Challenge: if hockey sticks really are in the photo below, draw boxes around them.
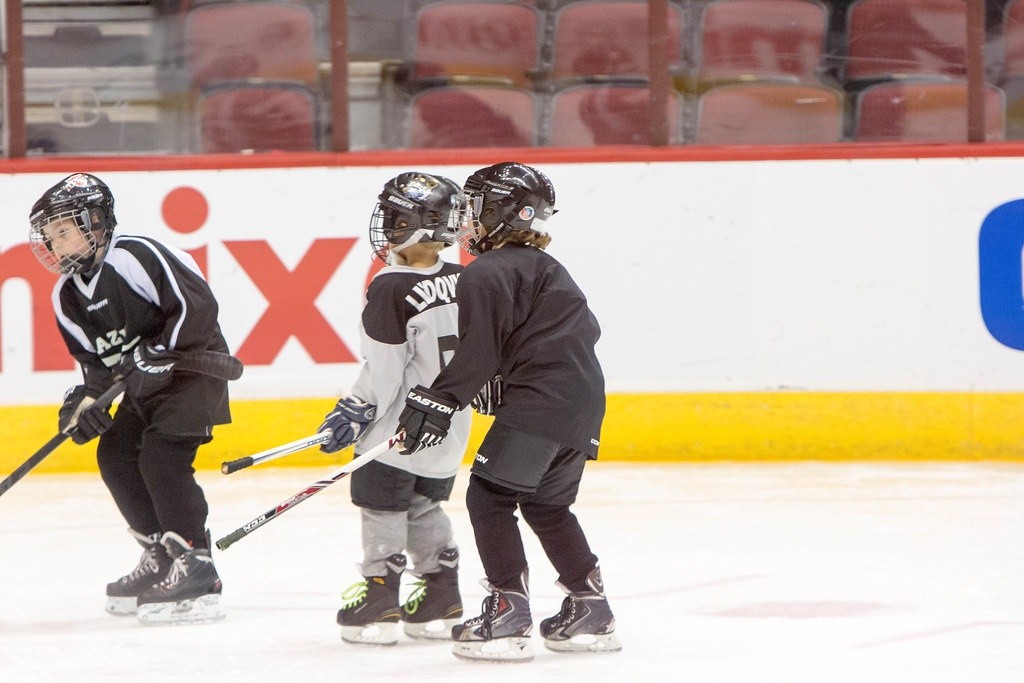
[221,431,331,474]
[214,434,404,548]
[0,349,242,498]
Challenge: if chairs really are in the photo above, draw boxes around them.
[168,0,1024,155]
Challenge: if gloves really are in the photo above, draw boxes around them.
[110,339,176,399]
[318,397,378,454]
[395,383,460,456]
[58,382,114,444]
[470,376,504,416]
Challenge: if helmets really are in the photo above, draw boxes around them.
[368,170,467,266]
[29,173,114,276]
[450,163,556,256]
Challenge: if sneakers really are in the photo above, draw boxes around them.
[450,569,534,661]
[335,553,408,646]
[105,528,176,616]
[398,548,464,640]
[540,564,622,650]
[136,526,226,626]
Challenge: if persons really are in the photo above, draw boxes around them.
[29,174,232,624]
[318,172,465,644]
[396,161,622,663]
